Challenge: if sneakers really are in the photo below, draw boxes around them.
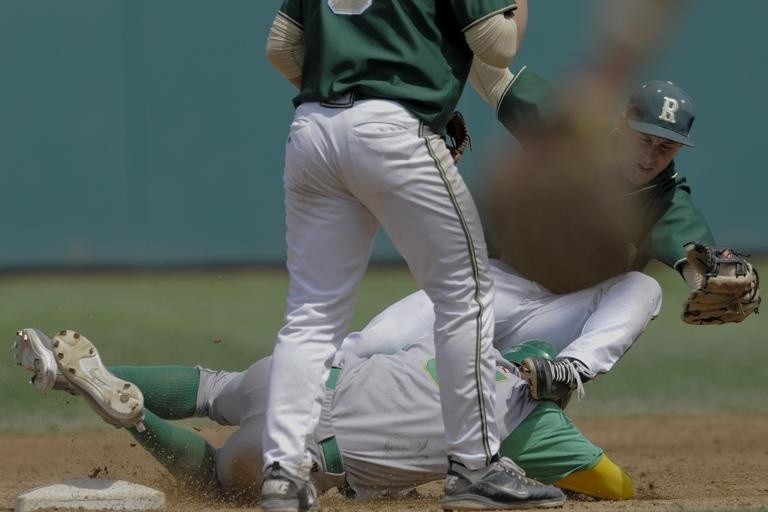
[259,460,317,511]
[522,357,596,401]
[51,329,146,433]
[13,327,70,394]
[441,454,567,512]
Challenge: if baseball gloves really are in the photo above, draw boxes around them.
[673,240,762,326]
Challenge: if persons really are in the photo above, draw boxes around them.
[340,52,759,400]
[258,0,568,512]
[12,327,636,499]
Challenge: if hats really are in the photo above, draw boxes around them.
[626,80,695,148]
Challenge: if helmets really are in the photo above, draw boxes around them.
[502,338,572,409]
